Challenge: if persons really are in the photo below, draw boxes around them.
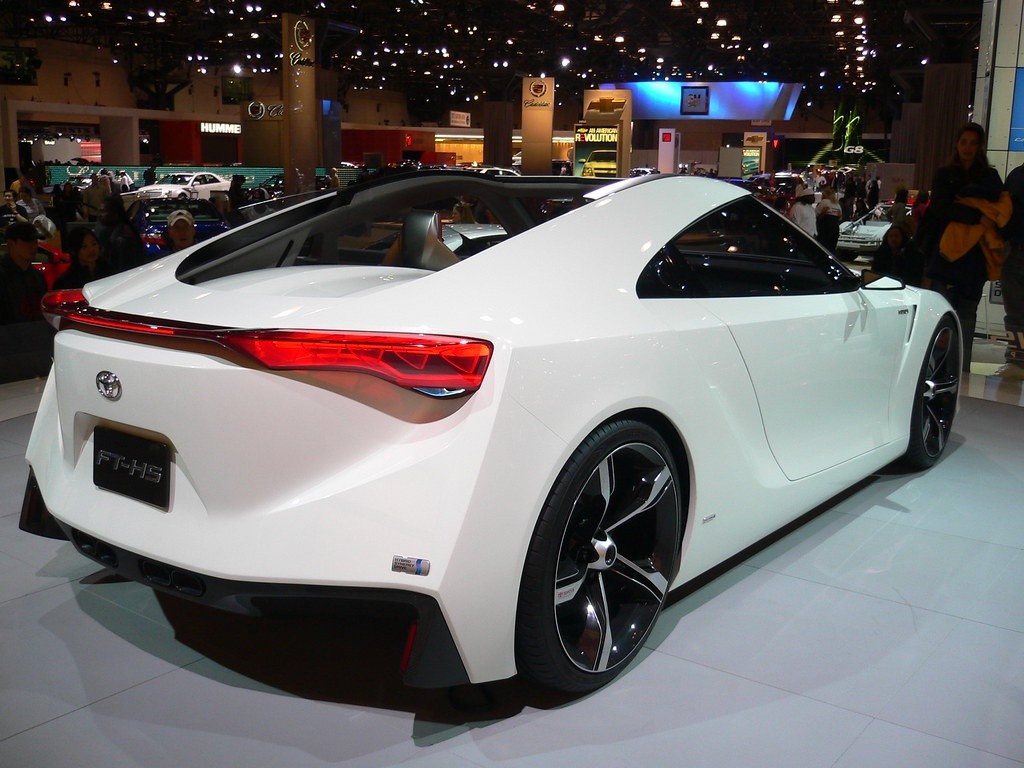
[912,123,1002,373]
[1000,166,1024,364]
[0,165,931,383]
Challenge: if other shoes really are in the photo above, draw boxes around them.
[1000,363,1024,380]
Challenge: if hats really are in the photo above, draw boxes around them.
[5,222,46,241]
[799,189,814,196]
[167,209,193,226]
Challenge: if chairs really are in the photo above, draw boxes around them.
[363,215,483,257]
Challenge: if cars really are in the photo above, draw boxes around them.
[833,201,917,264]
[465,165,522,178]
[631,167,657,177]
[577,149,618,180]
[722,170,808,211]
[134,172,233,201]
[1,170,332,210]
[127,197,231,260]
[28,239,74,289]
[333,204,511,266]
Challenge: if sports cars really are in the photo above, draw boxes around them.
[14,163,968,704]
